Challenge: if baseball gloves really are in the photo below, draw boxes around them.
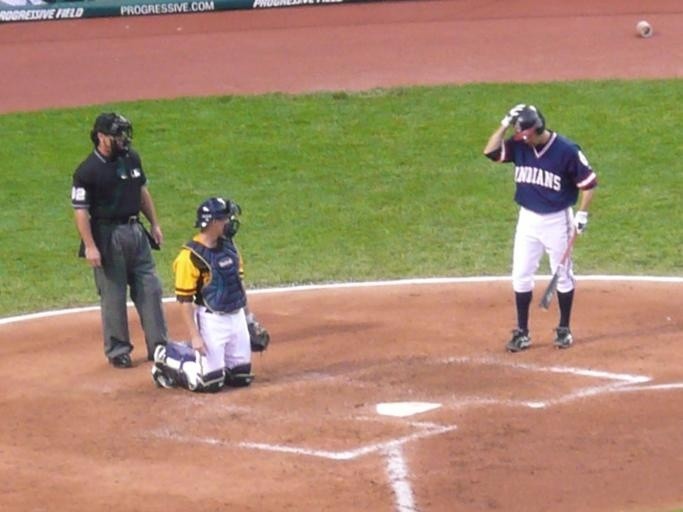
[246,318,268,352]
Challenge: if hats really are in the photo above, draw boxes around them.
[94,112,125,135]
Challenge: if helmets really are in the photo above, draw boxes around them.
[194,198,236,229]
[512,106,546,143]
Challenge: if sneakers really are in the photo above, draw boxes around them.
[508,326,533,351]
[553,326,574,349]
[106,350,133,370]
[152,344,168,388]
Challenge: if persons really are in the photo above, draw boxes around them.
[481,100,598,356]
[148,195,270,396]
[67,109,171,372]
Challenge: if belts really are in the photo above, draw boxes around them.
[92,216,140,224]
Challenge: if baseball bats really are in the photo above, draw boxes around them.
[538,227,579,312]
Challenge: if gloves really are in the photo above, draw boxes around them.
[572,210,588,231]
[500,102,526,127]
